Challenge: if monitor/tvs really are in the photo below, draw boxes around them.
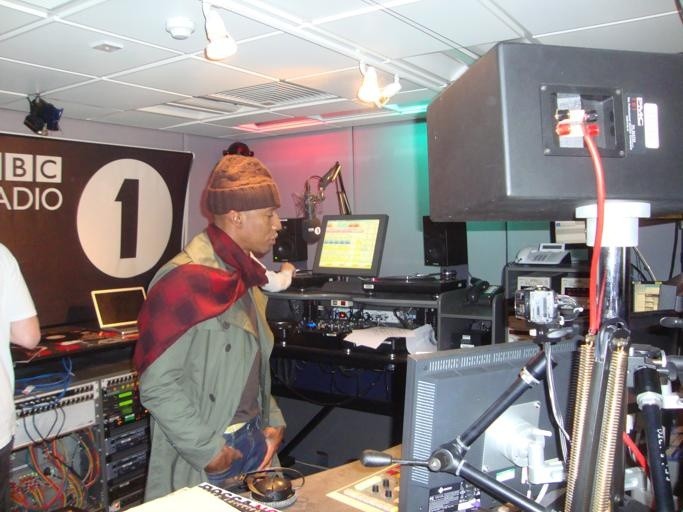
[312,214,388,292]
[398,339,578,512]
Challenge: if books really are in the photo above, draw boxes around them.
[122,480,282,512]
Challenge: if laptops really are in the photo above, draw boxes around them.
[91,287,147,334]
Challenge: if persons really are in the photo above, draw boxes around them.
[135,153,289,503]
[249,251,295,293]
[0,242,41,512]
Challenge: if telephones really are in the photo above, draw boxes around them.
[467,280,503,305]
[514,242,571,267]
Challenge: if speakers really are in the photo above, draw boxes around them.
[423,216,468,266]
[273,218,308,262]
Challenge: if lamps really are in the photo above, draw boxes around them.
[24,95,64,137]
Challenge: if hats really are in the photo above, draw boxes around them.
[206,154,280,215]
